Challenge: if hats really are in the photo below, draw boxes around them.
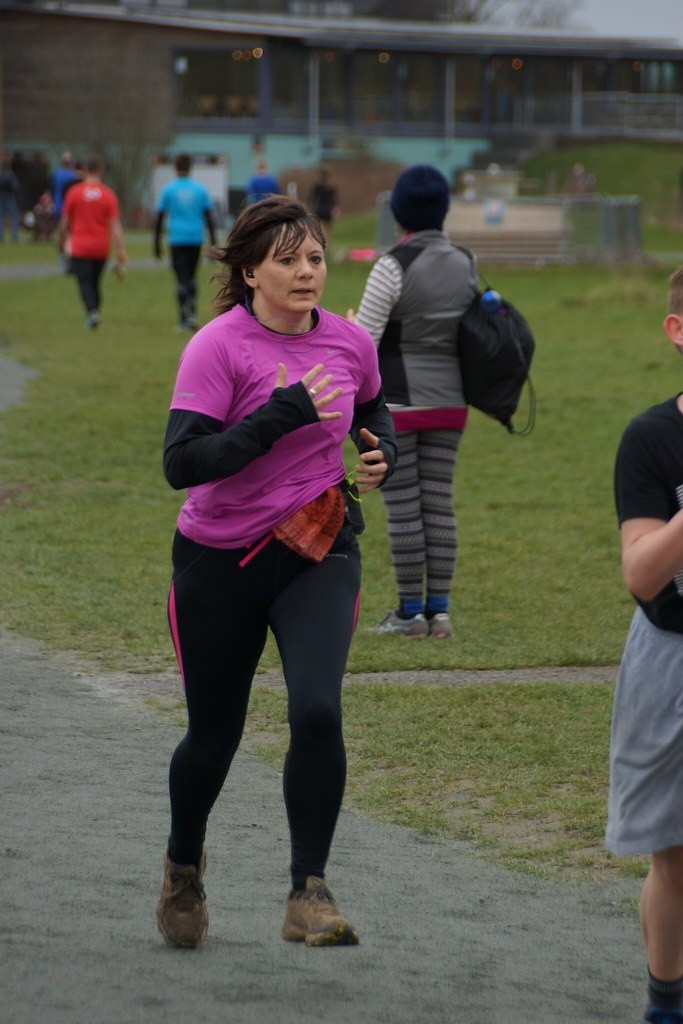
[390,166,449,231]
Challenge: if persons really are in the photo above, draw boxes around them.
[606,264,683,1024]
[347,166,482,638]
[0,151,127,330]
[155,196,400,951]
[246,159,280,206]
[152,149,230,332]
[304,170,341,249]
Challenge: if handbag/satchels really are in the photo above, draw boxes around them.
[455,260,536,437]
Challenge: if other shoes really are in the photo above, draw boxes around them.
[87,314,98,328]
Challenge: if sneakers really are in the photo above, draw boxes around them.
[282,875,359,946]
[367,607,428,639]
[428,613,453,638]
[157,847,209,949]
